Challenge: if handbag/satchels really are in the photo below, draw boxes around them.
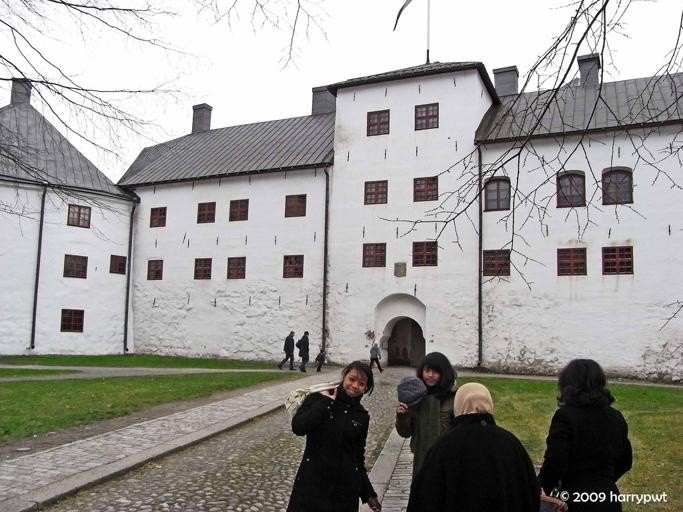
[536,471,570,512]
[284,379,342,418]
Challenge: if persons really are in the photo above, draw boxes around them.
[313,348,325,372]
[394,350,458,483]
[536,358,632,512]
[284,359,382,512]
[276,330,295,370]
[367,342,384,375]
[297,330,309,373]
[405,382,541,511]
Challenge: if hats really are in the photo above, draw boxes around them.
[397,376,429,407]
